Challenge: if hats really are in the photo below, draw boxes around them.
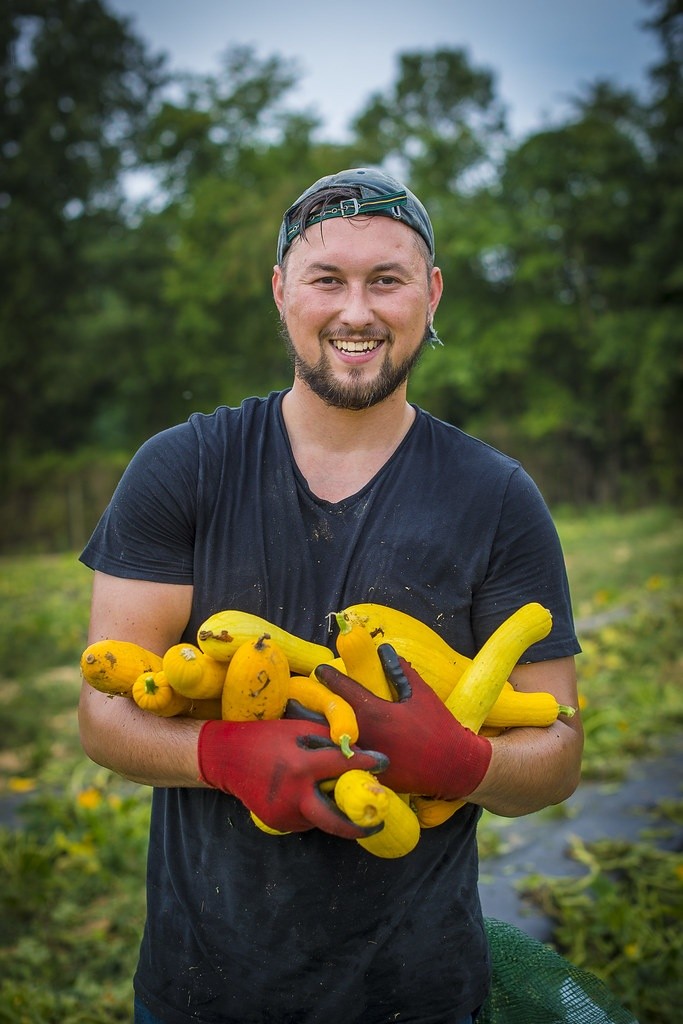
[276,168,435,340]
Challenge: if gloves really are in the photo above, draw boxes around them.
[198,718,387,839]
[282,645,491,801]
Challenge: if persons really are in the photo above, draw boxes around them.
[77,163,585,1022]
